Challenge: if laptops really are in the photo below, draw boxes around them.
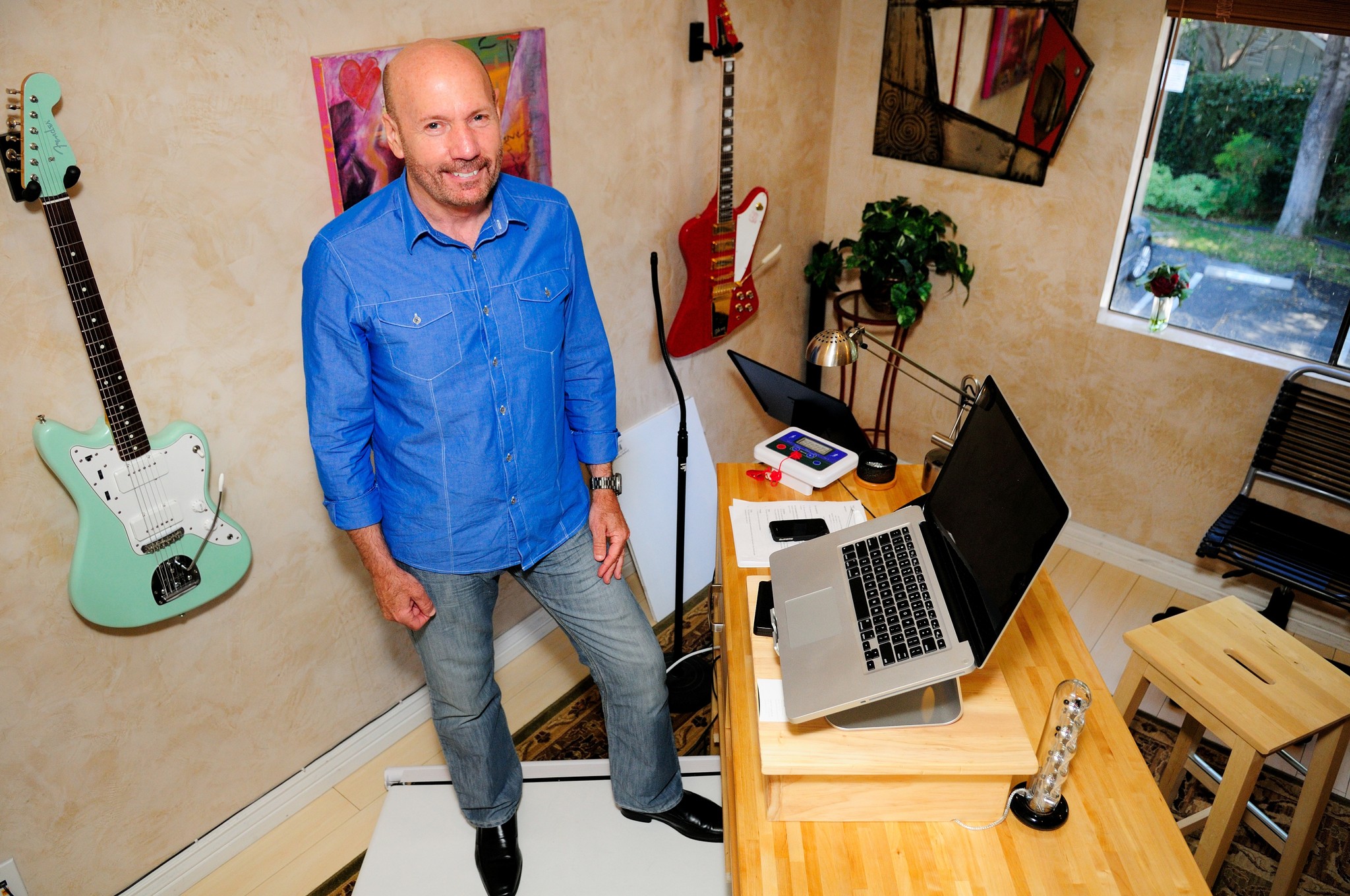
[768,374,1073,729]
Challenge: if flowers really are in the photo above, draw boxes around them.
[1135,261,1190,311]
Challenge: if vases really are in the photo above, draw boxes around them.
[1148,295,1175,332]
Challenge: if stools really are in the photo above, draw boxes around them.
[1111,592,1348,896]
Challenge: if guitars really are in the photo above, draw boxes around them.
[666,0,771,357]
[1,72,253,628]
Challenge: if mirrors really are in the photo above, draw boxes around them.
[870,0,1096,189]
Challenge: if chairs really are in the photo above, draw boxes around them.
[1145,365,1350,676]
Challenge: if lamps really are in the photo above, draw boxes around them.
[805,317,987,496]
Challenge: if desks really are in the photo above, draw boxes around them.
[713,461,1210,896]
[831,293,925,458]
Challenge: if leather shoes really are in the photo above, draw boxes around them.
[620,789,723,844]
[474,813,523,896]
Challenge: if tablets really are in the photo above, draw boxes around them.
[726,350,870,455]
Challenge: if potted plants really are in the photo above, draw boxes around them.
[800,196,976,333]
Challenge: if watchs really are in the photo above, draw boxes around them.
[588,472,623,498]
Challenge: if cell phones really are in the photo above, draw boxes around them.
[769,518,829,542]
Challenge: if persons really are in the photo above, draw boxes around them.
[300,38,723,896]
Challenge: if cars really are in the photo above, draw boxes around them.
[1115,213,1153,287]
